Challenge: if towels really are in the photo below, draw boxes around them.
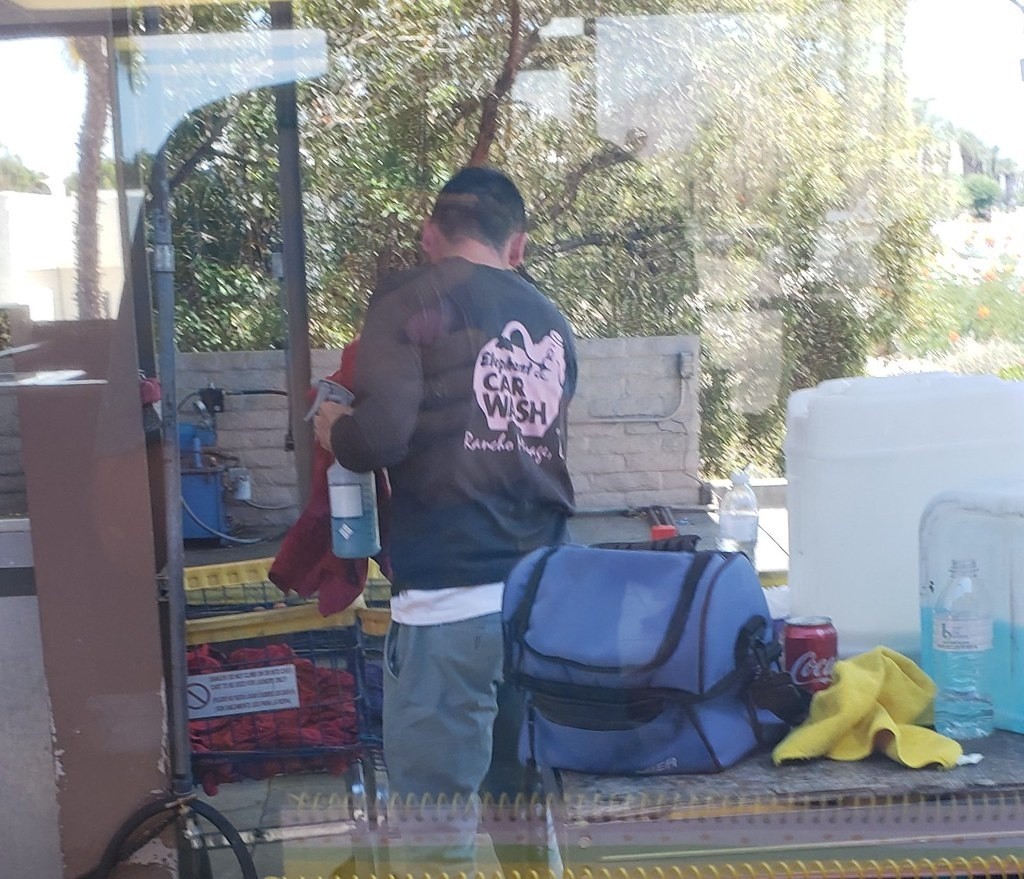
[187,643,358,795]
[268,340,395,614]
[773,644,983,771]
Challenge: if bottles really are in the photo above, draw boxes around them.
[716,472,760,566]
[327,467,380,559]
[933,560,996,740]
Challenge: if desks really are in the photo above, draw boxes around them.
[530,726,1024,879]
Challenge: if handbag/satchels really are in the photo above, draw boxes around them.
[500,545,813,777]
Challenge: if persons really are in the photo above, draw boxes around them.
[313,166,577,879]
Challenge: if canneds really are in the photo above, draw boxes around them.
[783,617,836,696]
